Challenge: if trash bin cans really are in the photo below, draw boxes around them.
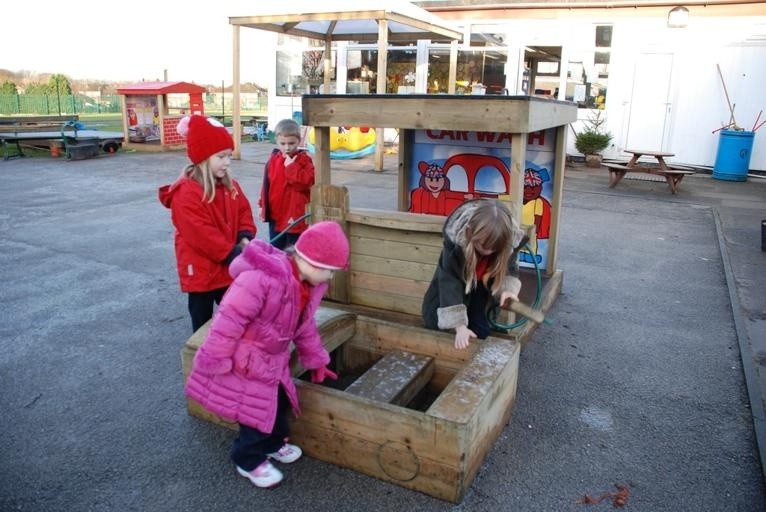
[711,129,755,182]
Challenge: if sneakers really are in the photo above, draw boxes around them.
[265,443,303,464]
[236,460,284,488]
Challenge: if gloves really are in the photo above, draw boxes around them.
[311,367,338,384]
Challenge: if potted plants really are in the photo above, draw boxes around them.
[575,132,613,168]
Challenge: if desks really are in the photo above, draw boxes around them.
[609,149,678,195]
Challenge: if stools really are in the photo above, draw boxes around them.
[600,159,695,195]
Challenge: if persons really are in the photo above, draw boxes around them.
[422,196,531,351]
[254,118,316,247]
[159,113,257,333]
[183,219,349,489]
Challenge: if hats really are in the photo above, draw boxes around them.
[294,221,350,272]
[176,115,234,164]
